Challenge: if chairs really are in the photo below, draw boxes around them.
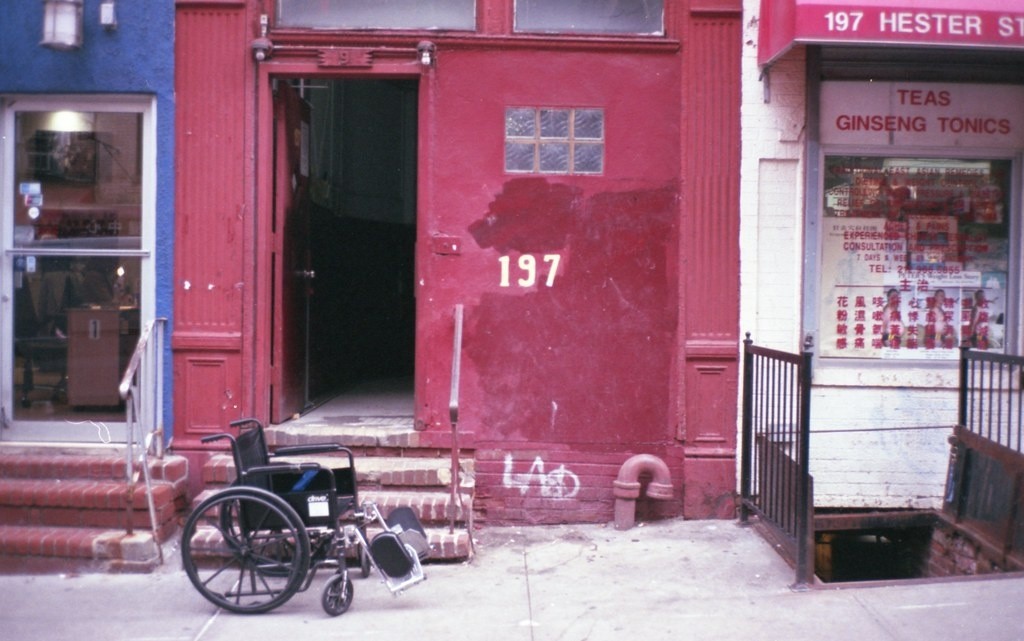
[16,270,107,409]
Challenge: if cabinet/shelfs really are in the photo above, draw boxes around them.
[63,303,139,411]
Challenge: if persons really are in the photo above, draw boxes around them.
[927,290,947,347]
[969,290,990,348]
[884,289,903,347]
[49,244,132,346]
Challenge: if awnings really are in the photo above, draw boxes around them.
[757,0,1024,102]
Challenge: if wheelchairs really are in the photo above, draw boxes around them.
[181,417,434,617]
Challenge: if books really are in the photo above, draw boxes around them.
[908,215,1008,274]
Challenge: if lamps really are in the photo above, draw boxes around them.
[40,0,83,49]
